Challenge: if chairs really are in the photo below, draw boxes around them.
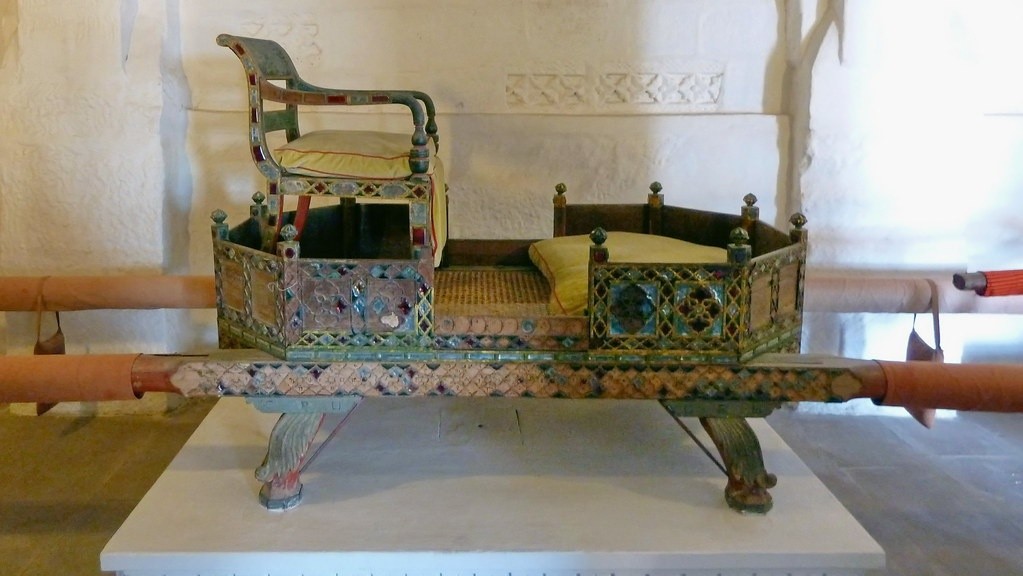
[218,31,448,269]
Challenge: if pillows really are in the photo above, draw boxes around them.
[530,231,727,315]
